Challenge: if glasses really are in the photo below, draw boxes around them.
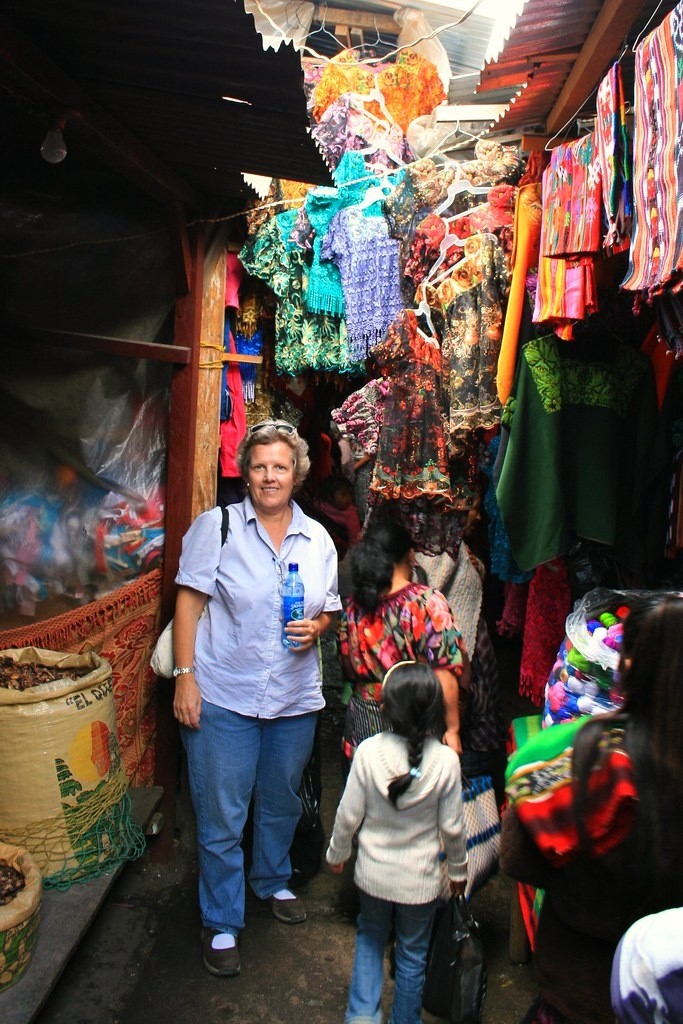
[250,423,294,440]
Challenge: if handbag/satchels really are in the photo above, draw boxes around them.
[149,601,209,677]
[387,889,485,1024]
[439,772,502,904]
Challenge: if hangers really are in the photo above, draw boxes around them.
[337,76,636,346]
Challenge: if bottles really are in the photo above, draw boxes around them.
[281,563,305,648]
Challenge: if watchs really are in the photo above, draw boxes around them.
[173,668,194,677]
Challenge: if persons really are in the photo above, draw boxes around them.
[172,421,342,975]
[337,520,464,782]
[327,661,468,1024]
[313,475,361,558]
[338,439,372,525]
[464,495,485,580]
[499,594,683,1023]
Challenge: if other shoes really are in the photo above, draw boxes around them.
[268,889,307,923]
[202,927,242,976]
